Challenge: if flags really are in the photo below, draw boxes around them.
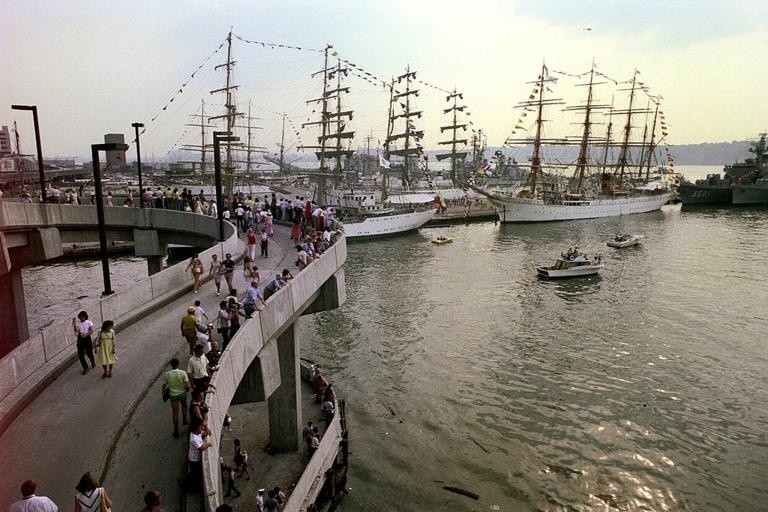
[379,155,392,170]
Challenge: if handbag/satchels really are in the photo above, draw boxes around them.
[216,327,229,334]
[163,387,170,402]
[99,486,113,511]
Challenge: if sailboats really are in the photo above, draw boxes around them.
[457,57,679,225]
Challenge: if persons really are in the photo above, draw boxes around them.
[72,310,119,379]
[303,420,322,465]
[309,362,337,431]
[10,479,58,512]
[437,236,447,240]
[561,247,579,260]
[140,489,167,512]
[616,233,630,241]
[73,470,112,512]
[19,186,345,296]
[161,344,250,512]
[180,279,268,368]
[256,486,286,512]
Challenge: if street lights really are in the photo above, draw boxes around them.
[90,143,130,296]
[11,104,50,205]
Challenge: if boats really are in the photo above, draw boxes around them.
[677,129,767,211]
[535,245,605,281]
[607,233,642,249]
